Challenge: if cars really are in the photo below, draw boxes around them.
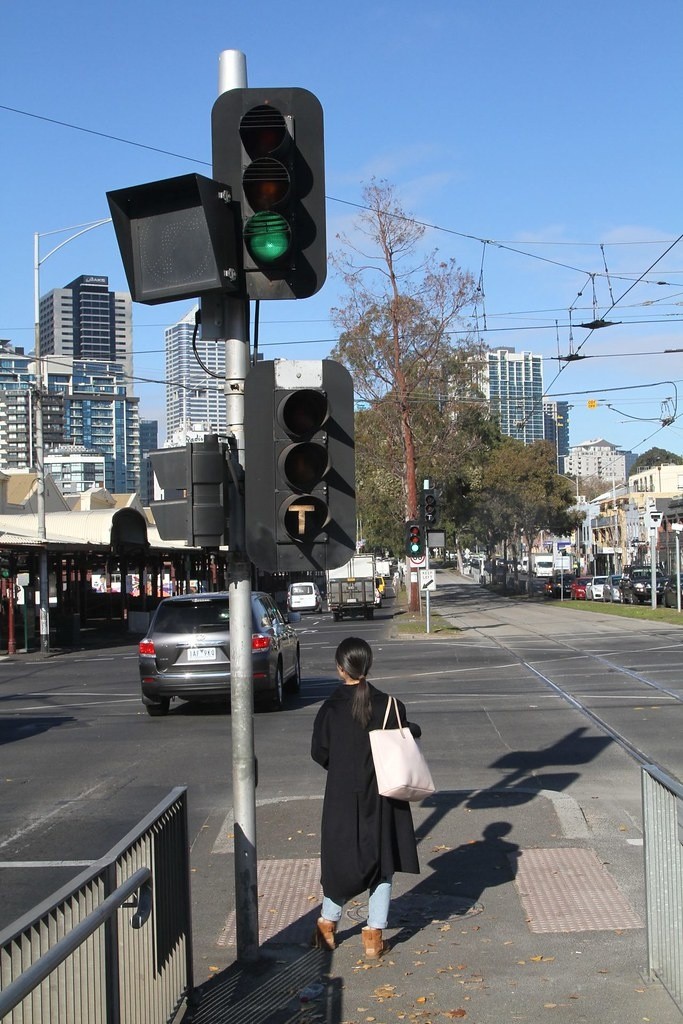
[374,576,387,609]
[442,551,521,573]
[543,572,683,609]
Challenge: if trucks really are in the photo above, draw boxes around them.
[327,552,376,619]
[376,560,392,581]
[521,555,553,577]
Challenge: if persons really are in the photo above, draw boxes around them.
[311,638,422,959]
[98,575,106,592]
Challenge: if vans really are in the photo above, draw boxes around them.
[288,583,323,613]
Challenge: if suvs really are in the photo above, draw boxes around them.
[137,591,302,714]
[620,566,670,604]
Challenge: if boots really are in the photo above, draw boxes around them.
[362,926,391,959]
[312,918,336,950]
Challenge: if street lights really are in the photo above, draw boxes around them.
[557,474,580,575]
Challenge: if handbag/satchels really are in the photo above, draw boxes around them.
[368,696,436,802]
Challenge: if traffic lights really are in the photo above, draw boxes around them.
[244,359,354,578]
[146,440,227,551]
[0,551,13,578]
[403,521,424,557]
[212,86,329,302]
[420,478,441,530]
[110,172,222,305]
